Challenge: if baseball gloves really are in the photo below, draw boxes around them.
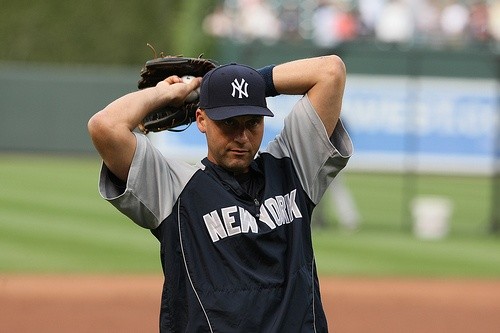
[135,43,220,136]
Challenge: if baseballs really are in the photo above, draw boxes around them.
[181,75,201,100]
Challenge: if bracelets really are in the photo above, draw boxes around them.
[254,65,279,99]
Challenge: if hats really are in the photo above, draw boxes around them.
[198,62,274,121]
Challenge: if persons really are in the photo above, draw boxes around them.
[85,54,354,333]
[202,0,500,56]
